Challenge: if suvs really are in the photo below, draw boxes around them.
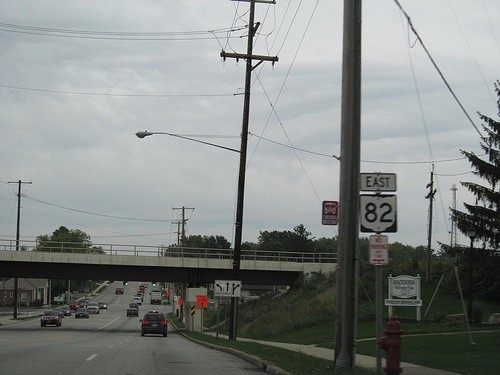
[140,311,169,337]
[85,302,101,314]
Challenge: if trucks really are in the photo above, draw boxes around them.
[150,291,162,305]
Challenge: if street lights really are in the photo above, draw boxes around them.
[136,129,247,341]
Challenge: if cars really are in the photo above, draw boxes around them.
[59,305,72,316]
[40,309,62,327]
[77,300,88,308]
[52,307,64,318]
[116,287,124,294]
[74,307,89,318]
[98,301,108,309]
[69,301,81,310]
[126,284,148,317]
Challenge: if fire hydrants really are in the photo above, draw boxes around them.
[377,317,406,375]
